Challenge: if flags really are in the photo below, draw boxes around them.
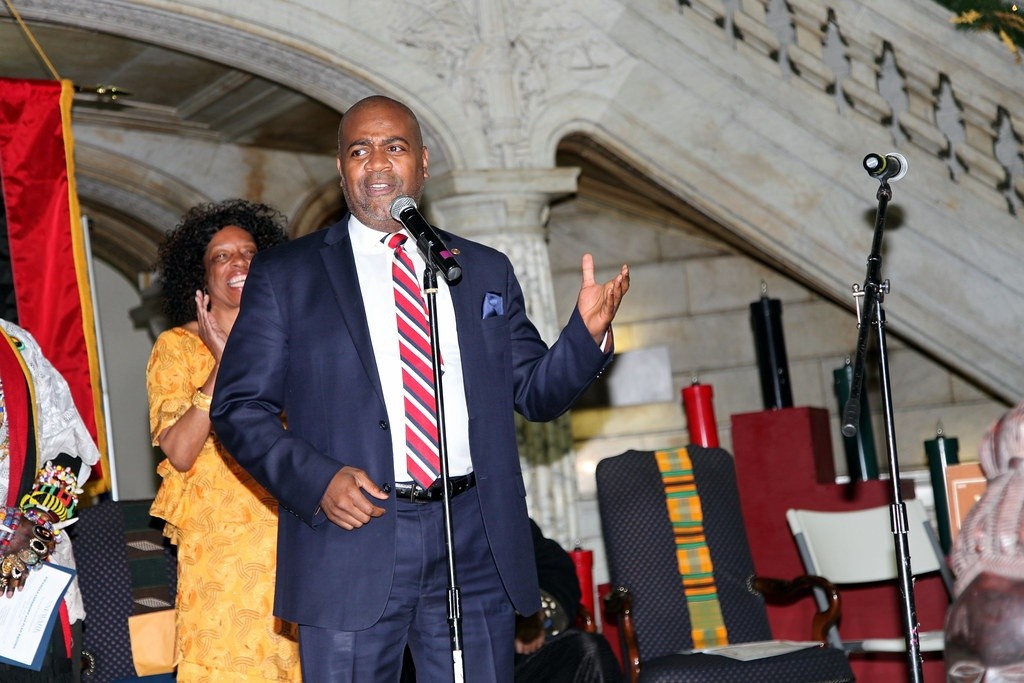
[1,76,111,498]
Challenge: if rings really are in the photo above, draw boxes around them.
[18,550,38,564]
[29,538,47,555]
[33,526,52,544]
[0,554,25,587]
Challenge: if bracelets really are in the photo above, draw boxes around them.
[19,461,78,544]
[0,506,22,554]
[193,387,213,412]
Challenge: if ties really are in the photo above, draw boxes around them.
[389,234,445,490]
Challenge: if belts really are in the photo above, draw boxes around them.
[396,471,476,502]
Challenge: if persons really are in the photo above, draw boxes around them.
[515,519,625,682]
[0,318,103,683]
[147,198,304,683]
[208,96,630,683]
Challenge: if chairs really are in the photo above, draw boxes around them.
[788,499,956,683]
[70,498,176,683]
[596,442,858,683]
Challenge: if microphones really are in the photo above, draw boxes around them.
[389,195,462,280]
[863,152,908,182]
